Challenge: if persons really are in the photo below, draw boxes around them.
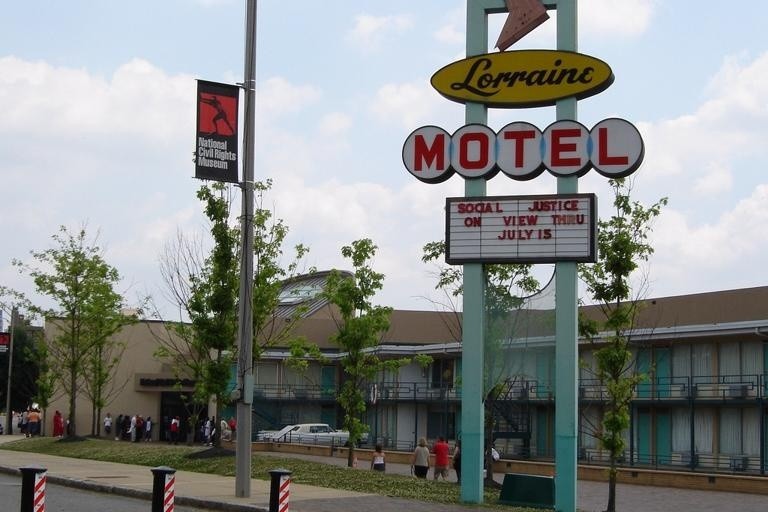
[370,445,386,474]
[410,438,431,480]
[431,436,450,482]
[199,93,234,134]
[103,412,237,447]
[51,410,63,439]
[451,440,460,483]
[17,408,42,438]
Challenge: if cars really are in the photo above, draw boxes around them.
[258,423,369,447]
